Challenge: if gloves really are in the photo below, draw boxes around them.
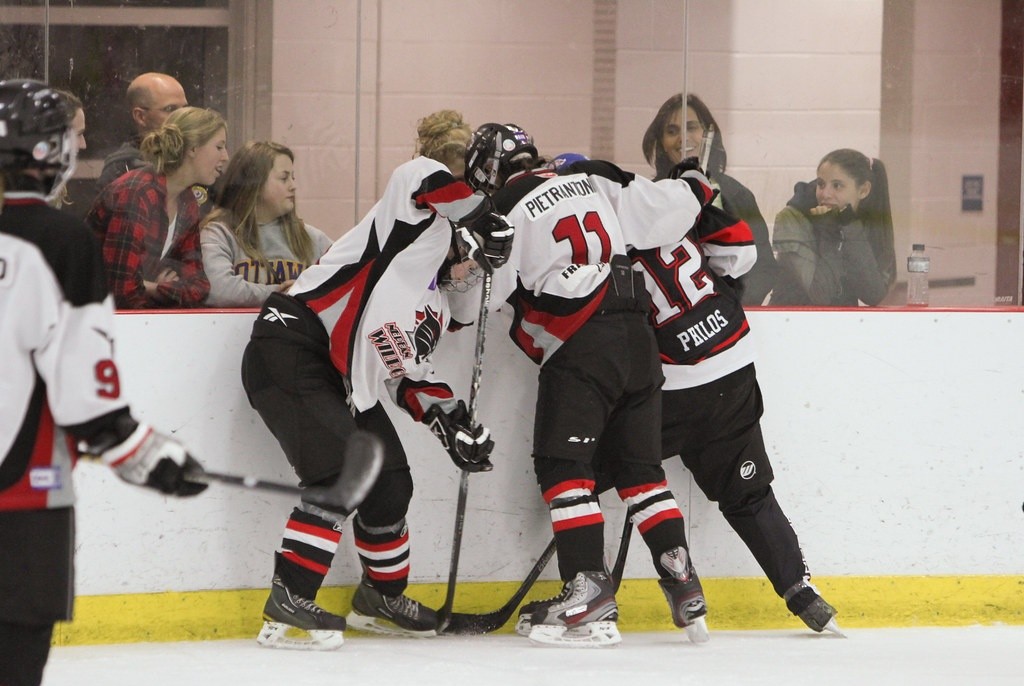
[447,188,515,275]
[117,431,207,498]
[421,400,495,473]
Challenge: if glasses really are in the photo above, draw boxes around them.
[140,105,188,115]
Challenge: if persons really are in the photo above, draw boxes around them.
[445,124,714,648]
[83,106,229,312]
[200,140,335,307]
[644,92,777,306]
[0,77,209,684]
[239,111,515,652]
[516,157,846,636]
[97,72,223,284]
[769,150,897,306]
[414,110,474,288]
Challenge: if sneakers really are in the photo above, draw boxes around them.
[530,555,622,648]
[515,578,574,636]
[255,551,346,651]
[346,556,440,637]
[798,594,847,640]
[656,546,709,645]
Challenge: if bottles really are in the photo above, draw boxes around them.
[906,244,930,307]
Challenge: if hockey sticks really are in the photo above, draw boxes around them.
[181,429,386,509]
[608,505,632,595]
[445,533,561,635]
[433,223,505,637]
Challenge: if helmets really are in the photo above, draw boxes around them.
[437,218,485,294]
[463,122,538,196]
[548,152,589,173]
[0,79,69,163]
